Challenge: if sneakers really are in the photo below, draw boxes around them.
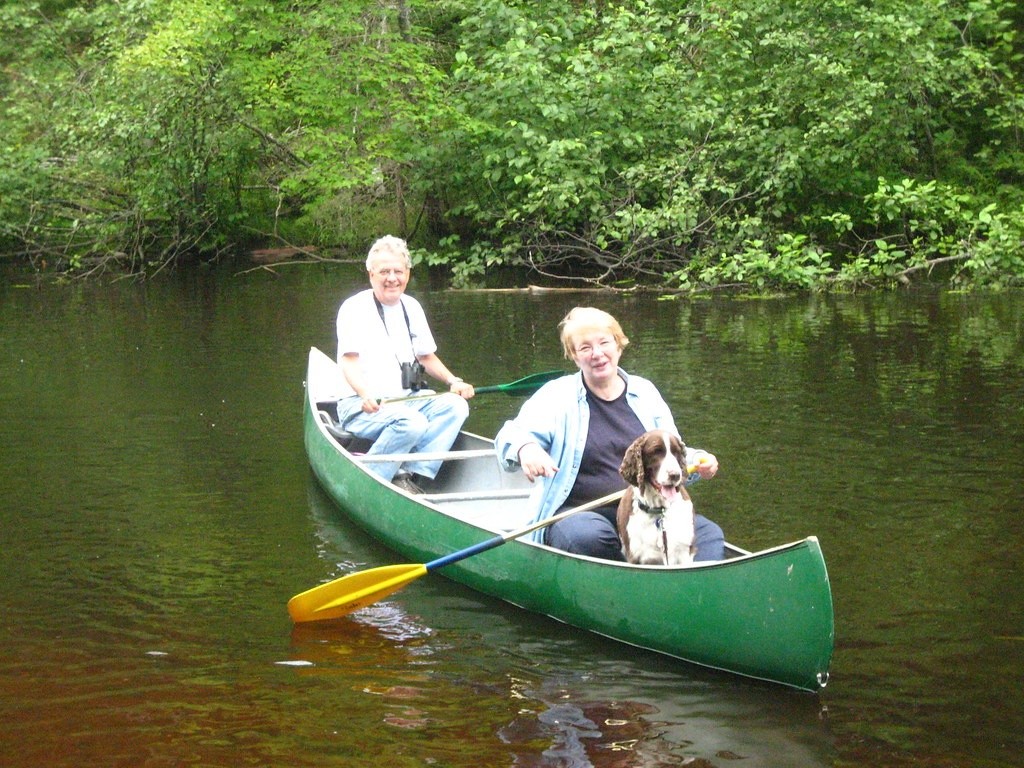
[391,473,426,495]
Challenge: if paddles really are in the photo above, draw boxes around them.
[286,460,700,621]
[374,368,567,404]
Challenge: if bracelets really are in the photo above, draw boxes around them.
[449,377,463,384]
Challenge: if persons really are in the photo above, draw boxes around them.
[336,234,475,495]
[495,307,724,561]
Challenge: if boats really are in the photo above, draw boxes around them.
[301,346,833,694]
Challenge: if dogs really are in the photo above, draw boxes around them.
[615,429,696,565]
